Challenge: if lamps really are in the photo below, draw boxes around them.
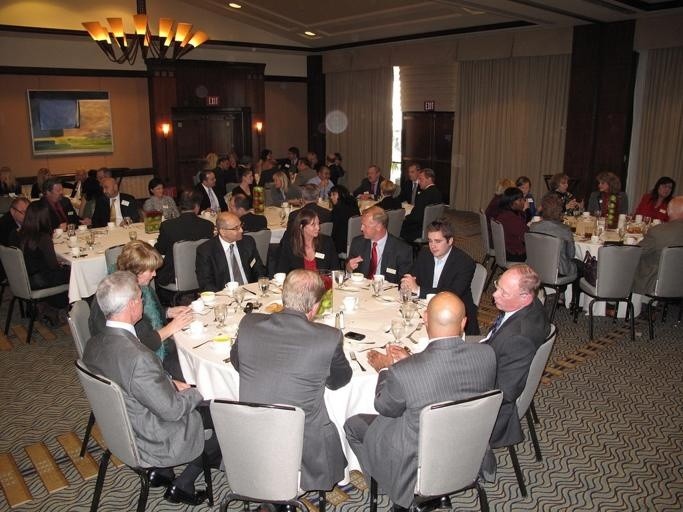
[256,118,264,161]
[161,119,170,185]
[82,0,209,65]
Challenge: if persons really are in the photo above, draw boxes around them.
[344,163,479,335]
[193,148,361,295]
[1,165,139,326]
[480,264,551,451]
[484,172,683,321]
[142,178,180,221]
[230,268,352,511]
[343,290,497,512]
[154,189,215,306]
[82,271,223,505]
[87,239,198,383]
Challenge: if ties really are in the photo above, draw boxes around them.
[412,183,416,204]
[109,200,116,222]
[229,244,244,286]
[370,241,378,277]
[208,189,216,213]
[371,183,374,195]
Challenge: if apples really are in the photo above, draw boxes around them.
[317,275,332,314]
[608,195,617,226]
[145,216,161,232]
[253,186,264,213]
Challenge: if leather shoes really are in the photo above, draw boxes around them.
[163,485,208,505]
[146,466,176,489]
[260,502,298,512]
[387,495,452,512]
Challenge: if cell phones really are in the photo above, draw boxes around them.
[345,331,365,341]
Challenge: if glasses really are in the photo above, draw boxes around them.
[222,222,245,231]
[492,279,522,299]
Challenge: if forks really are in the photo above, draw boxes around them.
[350,352,367,373]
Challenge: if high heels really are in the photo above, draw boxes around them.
[41,310,65,328]
[569,303,583,315]
[556,298,567,309]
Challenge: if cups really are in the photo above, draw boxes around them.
[279,201,294,226]
[53,216,157,258]
[181,268,439,353]
[565,211,663,245]
[532,215,543,222]
[205,207,221,220]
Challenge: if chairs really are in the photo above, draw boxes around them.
[370,389,503,512]
[507,324,558,497]
[209,399,311,512]
[75,358,214,512]
[68,299,95,458]
